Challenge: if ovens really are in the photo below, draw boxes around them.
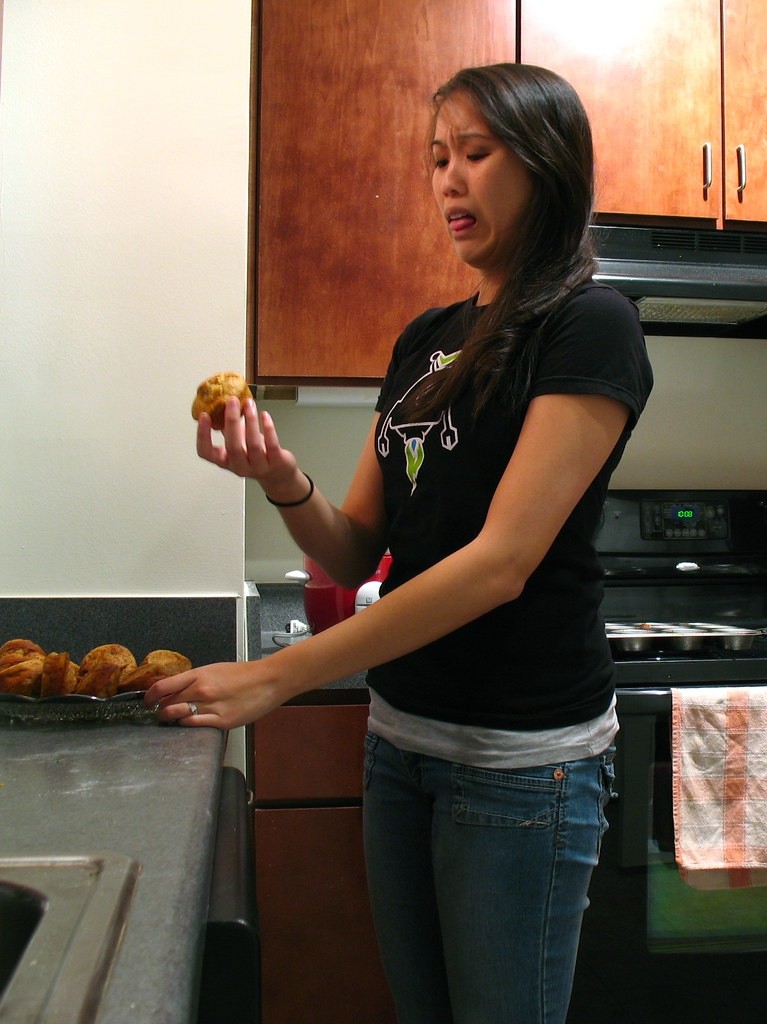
[566,686,766,1024]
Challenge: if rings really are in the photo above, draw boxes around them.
[188,702,199,715]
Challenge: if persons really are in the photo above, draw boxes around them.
[142,63,655,1024]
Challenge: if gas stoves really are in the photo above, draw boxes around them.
[591,489,766,683]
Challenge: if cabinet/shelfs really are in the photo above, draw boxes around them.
[252,702,394,1024]
[251,0,767,388]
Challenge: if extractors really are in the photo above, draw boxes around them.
[587,225,767,339]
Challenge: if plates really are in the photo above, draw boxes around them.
[0,690,161,731]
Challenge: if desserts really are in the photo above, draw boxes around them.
[0,637,191,699]
[192,372,254,430]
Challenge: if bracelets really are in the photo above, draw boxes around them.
[266,472,314,508]
